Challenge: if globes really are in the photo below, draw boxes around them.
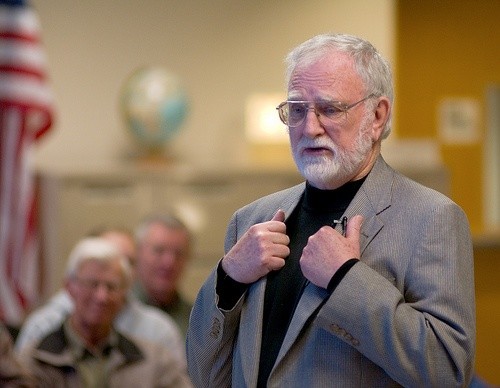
[115,66,189,171]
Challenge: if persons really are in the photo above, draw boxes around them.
[186,31,475,388]
[16,223,187,388]
[131,211,192,334]
[15,239,193,388]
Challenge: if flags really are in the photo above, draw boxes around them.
[0,0,60,388]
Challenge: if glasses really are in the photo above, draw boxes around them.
[278,95,372,129]
